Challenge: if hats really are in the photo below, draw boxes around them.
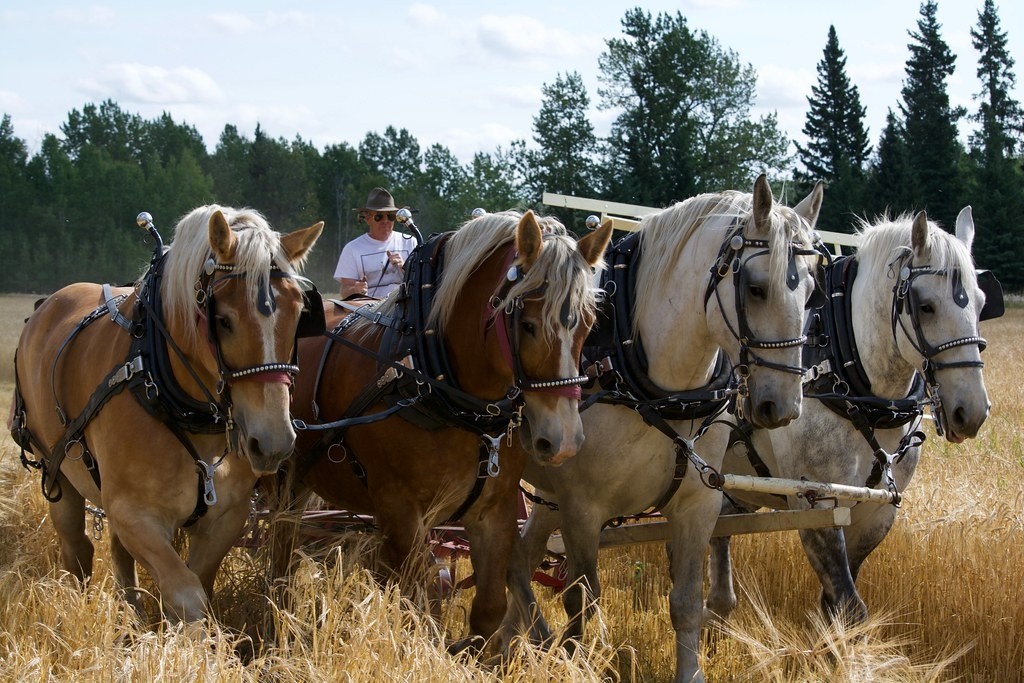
[351,187,399,213]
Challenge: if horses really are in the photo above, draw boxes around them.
[6,199,328,649]
[248,205,615,670]
[662,202,992,682]
[478,172,825,682]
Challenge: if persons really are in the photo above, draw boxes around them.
[333,187,418,300]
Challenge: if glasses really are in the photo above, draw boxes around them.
[367,213,396,222]
